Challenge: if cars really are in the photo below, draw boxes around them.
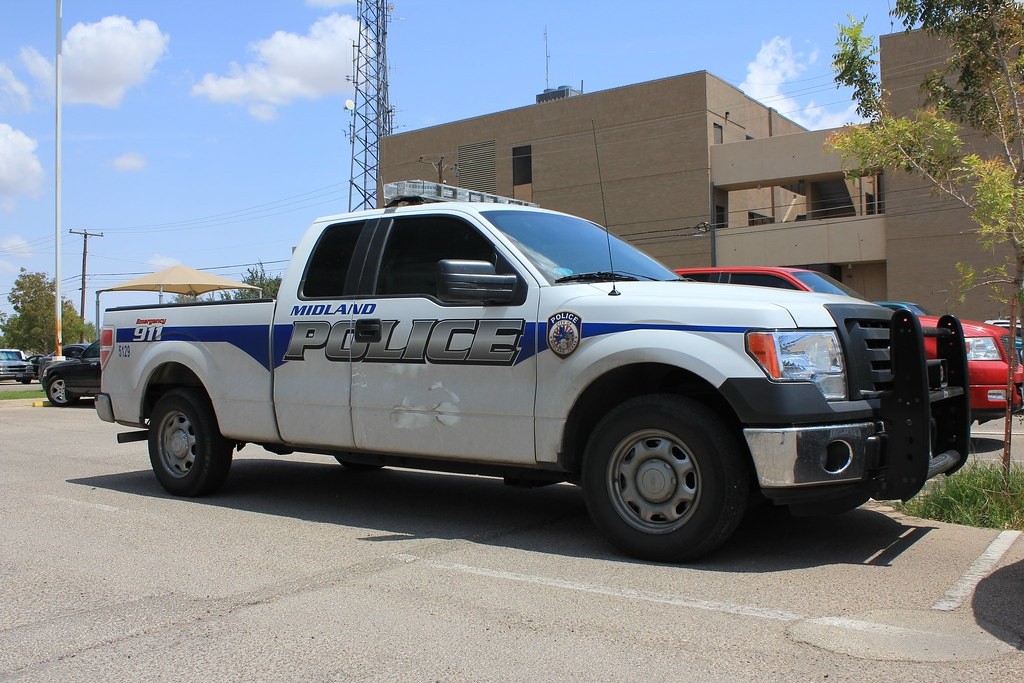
[26,355,45,378]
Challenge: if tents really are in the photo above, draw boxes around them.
[95,265,262,339]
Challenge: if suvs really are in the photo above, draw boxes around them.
[39,339,101,407]
[0,349,35,384]
[673,269,1024,424]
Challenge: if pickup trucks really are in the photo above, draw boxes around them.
[96,180,970,562]
